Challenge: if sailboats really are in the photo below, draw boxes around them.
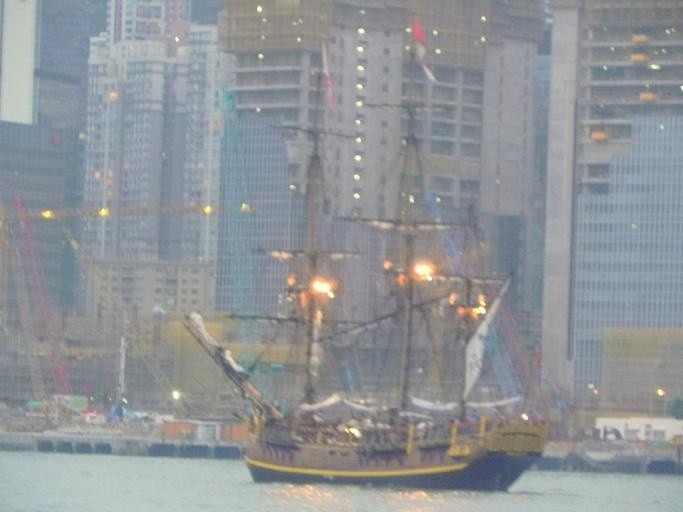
[179,15,555,494]
